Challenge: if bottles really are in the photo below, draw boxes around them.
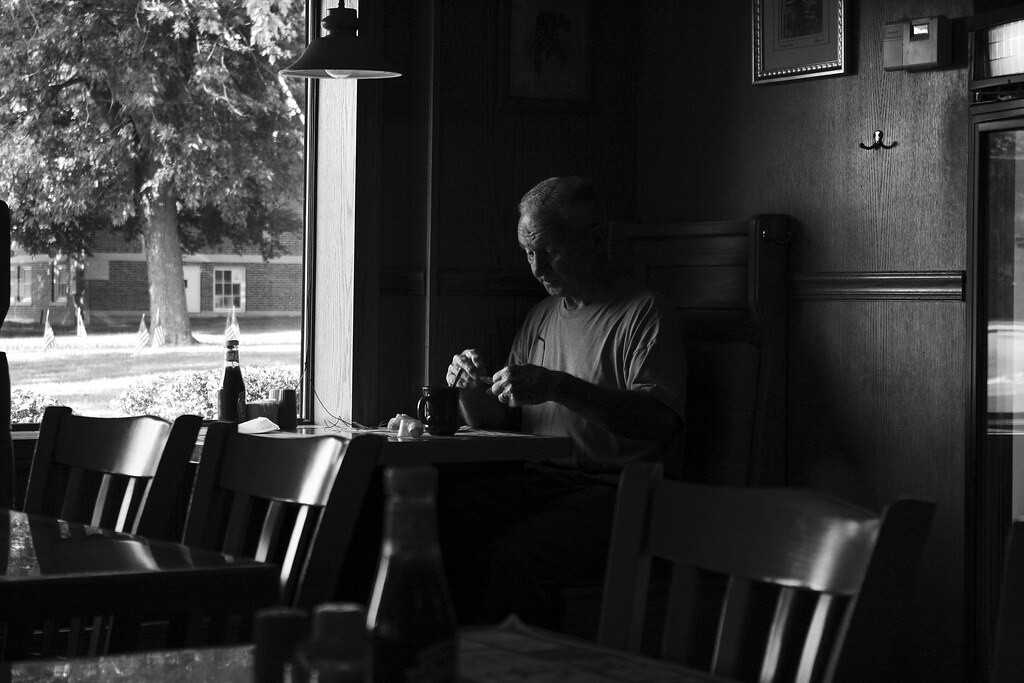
[222,340,247,425]
[251,602,366,683]
[368,468,458,683]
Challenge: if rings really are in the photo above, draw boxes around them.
[500,393,507,402]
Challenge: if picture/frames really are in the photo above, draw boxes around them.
[498,0,597,112]
[752,0,848,87]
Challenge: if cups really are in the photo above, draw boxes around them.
[418,386,462,437]
[269,390,297,428]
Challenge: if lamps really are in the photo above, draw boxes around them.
[286,0,402,80]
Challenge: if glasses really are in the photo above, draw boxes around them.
[477,336,546,385]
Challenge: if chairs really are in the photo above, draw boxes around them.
[506,211,799,623]
[180,423,361,603]
[594,462,936,683]
[19,404,201,661]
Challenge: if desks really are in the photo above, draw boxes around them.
[0,508,276,658]
[6,615,743,683]
[179,429,572,608]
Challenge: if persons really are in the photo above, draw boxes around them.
[415,175,685,655]
